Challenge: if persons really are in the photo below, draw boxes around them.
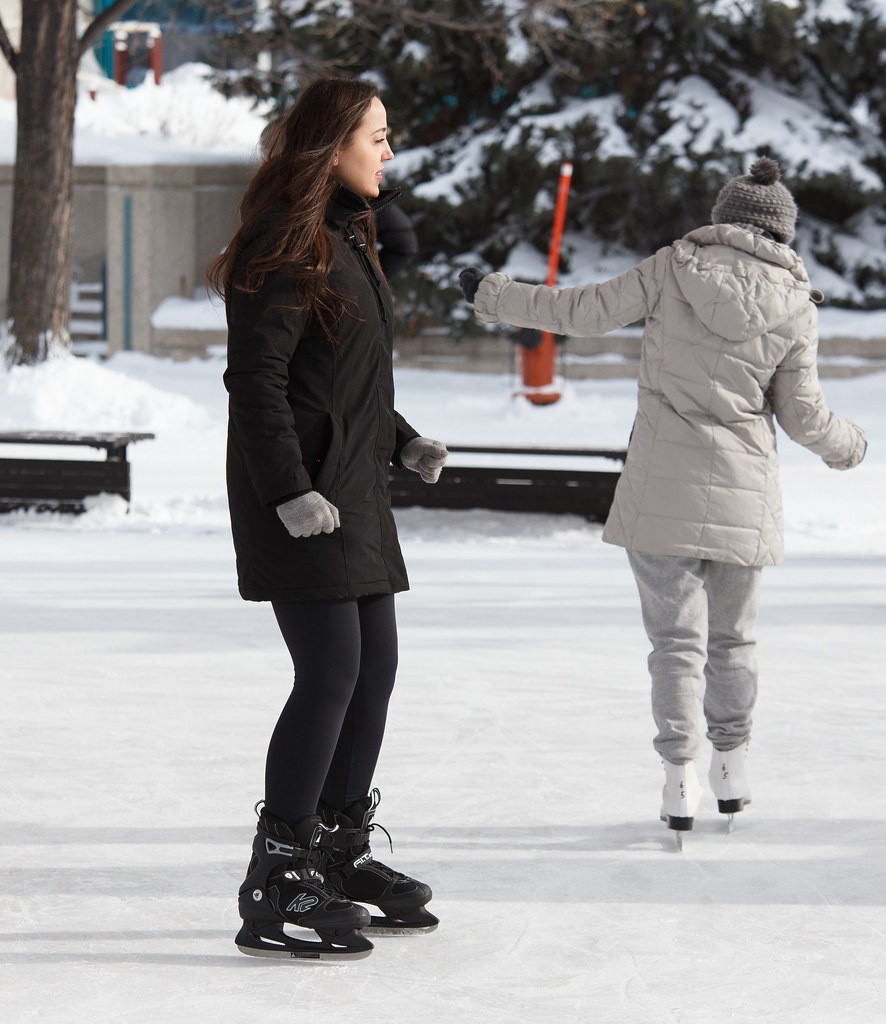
[203,78,448,962]
[459,154,866,851]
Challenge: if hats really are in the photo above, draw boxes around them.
[711,155,797,244]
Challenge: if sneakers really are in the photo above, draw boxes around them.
[313,787,440,937]
[708,739,752,832]
[660,759,702,851]
[233,800,374,960]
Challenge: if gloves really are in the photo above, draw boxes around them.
[401,437,450,483]
[277,491,341,538]
[459,266,483,303]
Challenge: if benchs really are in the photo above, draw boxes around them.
[0,428,153,519]
[389,446,628,529]
